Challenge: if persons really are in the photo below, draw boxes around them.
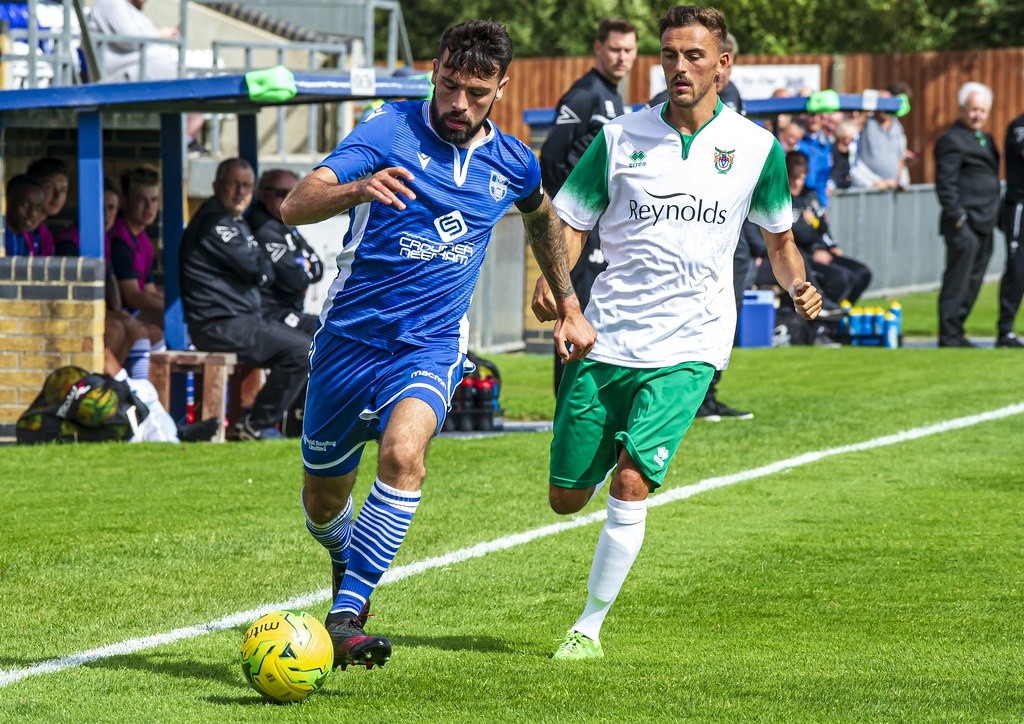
[280,19,597,673]
[4,156,229,443]
[85,0,223,83]
[177,158,324,443]
[530,4,824,663]
[742,80,921,347]
[932,81,1024,349]
[539,19,755,426]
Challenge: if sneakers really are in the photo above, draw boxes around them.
[694,385,754,422]
[323,520,391,672]
[550,629,605,661]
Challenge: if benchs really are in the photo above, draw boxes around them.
[148,350,256,444]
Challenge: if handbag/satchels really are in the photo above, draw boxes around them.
[16,367,149,445]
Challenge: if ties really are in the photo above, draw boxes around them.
[974,130,987,147]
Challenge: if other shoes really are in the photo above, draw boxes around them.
[177,417,219,442]
[813,333,841,348]
[939,336,975,347]
[996,333,1024,347]
[230,413,265,442]
[810,292,842,318]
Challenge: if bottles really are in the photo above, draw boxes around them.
[840,299,903,349]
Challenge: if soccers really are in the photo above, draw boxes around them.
[16,363,130,443]
[241,609,335,704]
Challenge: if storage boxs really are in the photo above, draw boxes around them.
[737,290,775,348]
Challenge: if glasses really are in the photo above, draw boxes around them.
[264,186,290,198]
